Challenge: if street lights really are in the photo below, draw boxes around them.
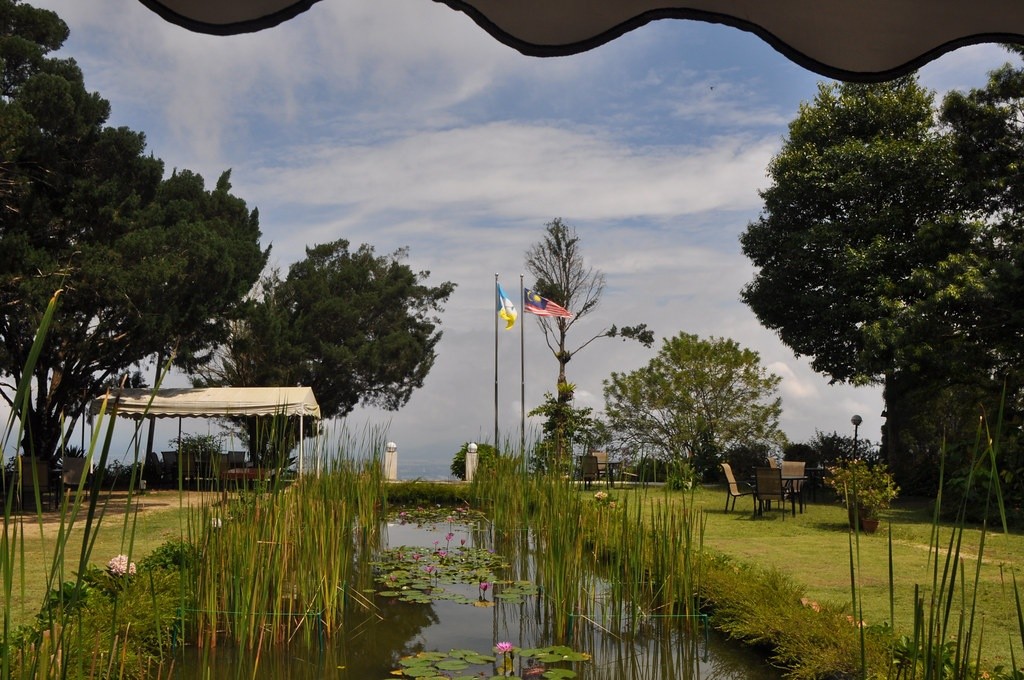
[851,415,862,458]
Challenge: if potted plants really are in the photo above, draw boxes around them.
[823,456,901,534]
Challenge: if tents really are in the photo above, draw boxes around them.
[89,386,320,483]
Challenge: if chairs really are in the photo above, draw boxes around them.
[580,451,608,491]
[21,457,87,512]
[151,451,245,491]
[719,456,806,521]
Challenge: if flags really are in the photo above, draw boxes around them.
[496,284,517,330]
[524,288,573,318]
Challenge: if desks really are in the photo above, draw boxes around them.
[174,460,212,490]
[578,463,622,488]
[804,468,825,502]
[8,470,69,510]
[752,475,809,518]
